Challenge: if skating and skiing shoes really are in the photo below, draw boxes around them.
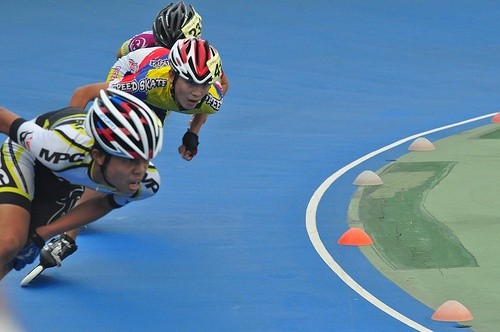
[19,232,78,288]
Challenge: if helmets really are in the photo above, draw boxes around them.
[84,86,164,162]
[167,36,223,84]
[152,0,203,51]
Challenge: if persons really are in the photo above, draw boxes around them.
[0,88,165,282]
[36,36,226,268]
[116,0,207,64]
